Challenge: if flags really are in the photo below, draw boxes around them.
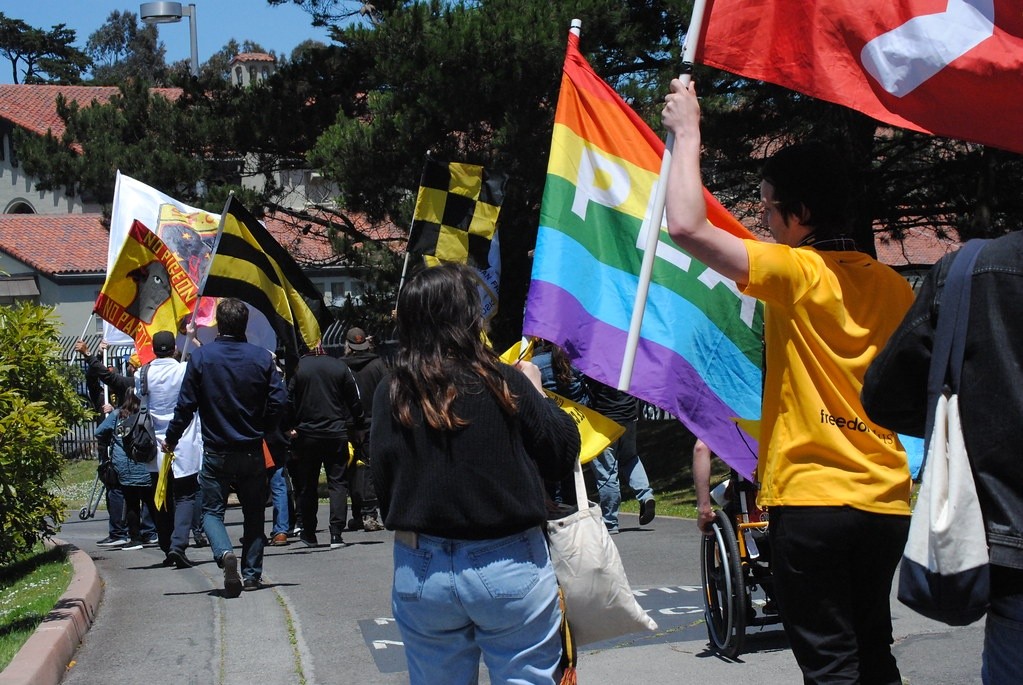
[407,155,509,271]
[154,451,173,513]
[201,191,333,357]
[105,173,222,345]
[523,35,763,484]
[502,344,627,466]
[699,0,1022,148]
[94,219,200,366]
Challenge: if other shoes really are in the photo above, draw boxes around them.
[363,515,384,531]
[140,532,161,547]
[731,596,757,618]
[121,538,143,550]
[169,548,192,569]
[162,556,176,566]
[222,550,243,597]
[269,532,288,546]
[194,532,208,547]
[244,576,264,590]
[348,514,364,529]
[331,533,346,549]
[763,599,783,614]
[638,497,655,525]
[300,532,317,547]
[96,533,131,546]
[606,526,619,534]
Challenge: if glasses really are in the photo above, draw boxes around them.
[126,361,130,367]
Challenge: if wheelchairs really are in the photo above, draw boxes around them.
[699,470,784,660]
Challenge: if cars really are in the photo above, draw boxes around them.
[71,379,93,411]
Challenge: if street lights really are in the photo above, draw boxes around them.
[140,1,206,202]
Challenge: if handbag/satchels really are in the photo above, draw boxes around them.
[546,453,659,648]
[897,239,995,626]
[96,461,121,490]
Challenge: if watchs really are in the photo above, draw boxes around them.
[84,352,91,357]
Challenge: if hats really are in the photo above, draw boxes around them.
[153,331,175,352]
[129,352,141,367]
[346,327,369,351]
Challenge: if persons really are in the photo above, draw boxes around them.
[661,78,915,685]
[861,227,1023,685]
[161,299,287,598]
[75,330,207,568]
[262,440,289,545]
[368,264,580,685]
[532,334,655,533]
[692,439,778,613]
[287,328,390,548]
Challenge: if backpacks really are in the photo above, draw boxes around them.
[122,364,158,464]
